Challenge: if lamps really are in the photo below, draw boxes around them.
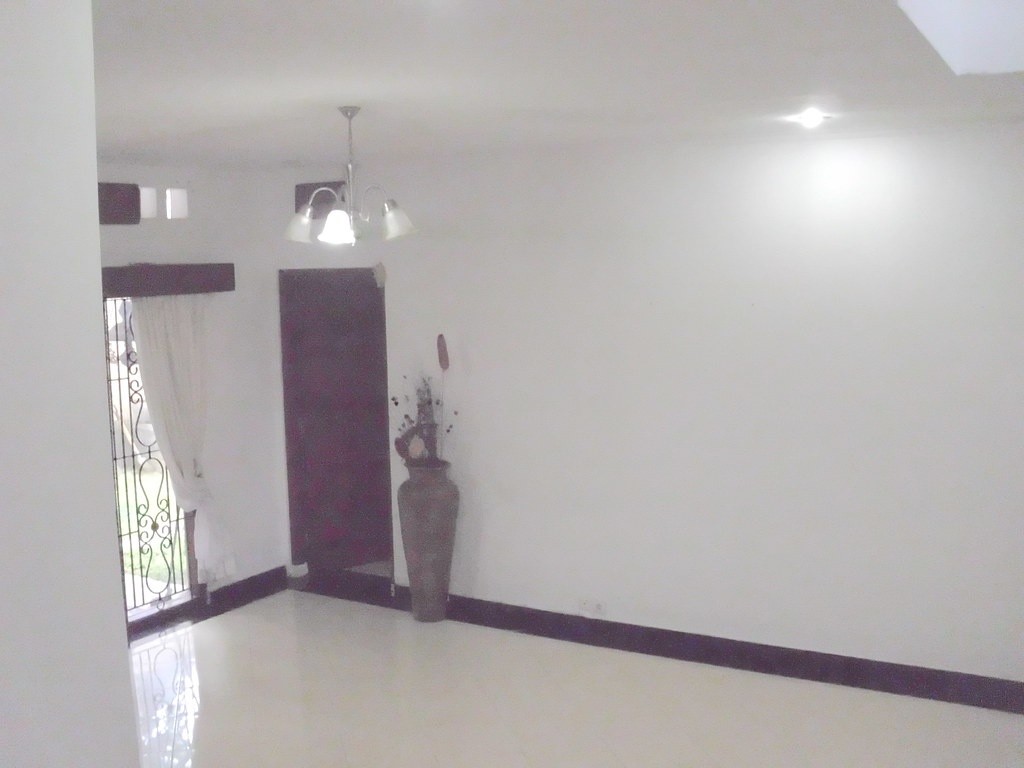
[283,106,420,246]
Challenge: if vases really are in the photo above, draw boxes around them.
[397,459,460,622]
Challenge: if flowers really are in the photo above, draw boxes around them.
[391,334,459,467]
